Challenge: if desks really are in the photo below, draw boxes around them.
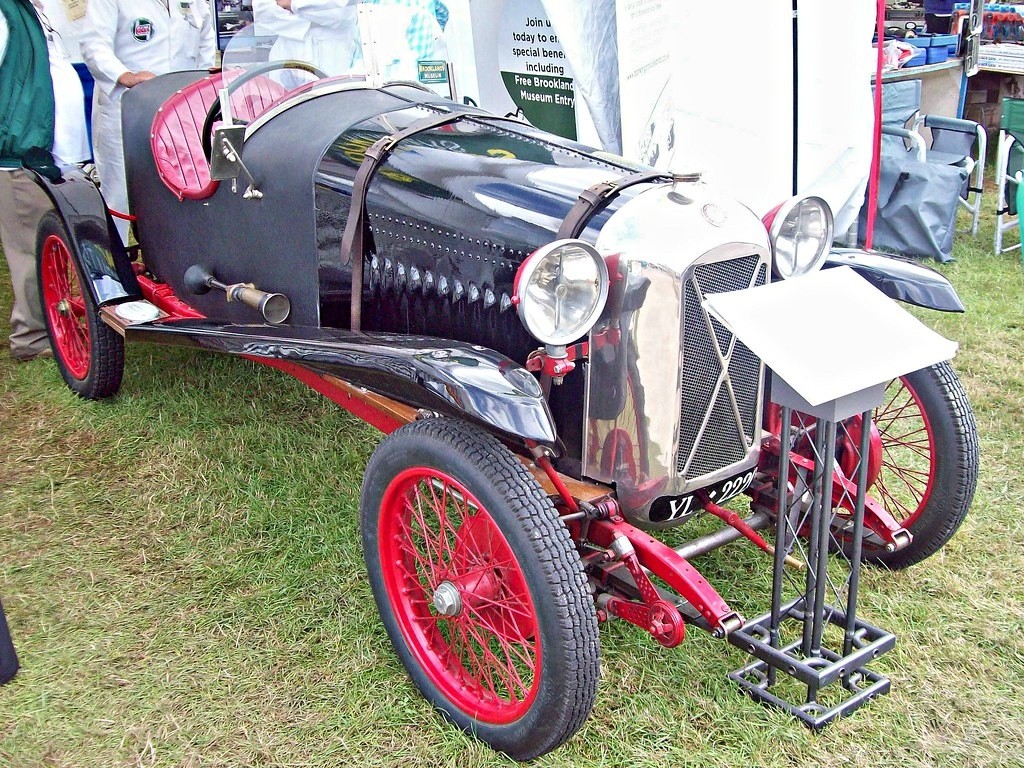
[870,56,968,151]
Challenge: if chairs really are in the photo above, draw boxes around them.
[994,97,1024,257]
[906,113,987,237]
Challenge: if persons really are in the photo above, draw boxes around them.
[0,0,364,363]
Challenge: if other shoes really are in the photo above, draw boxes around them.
[19,347,55,362]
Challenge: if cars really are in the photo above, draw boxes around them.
[34,32,981,766]
